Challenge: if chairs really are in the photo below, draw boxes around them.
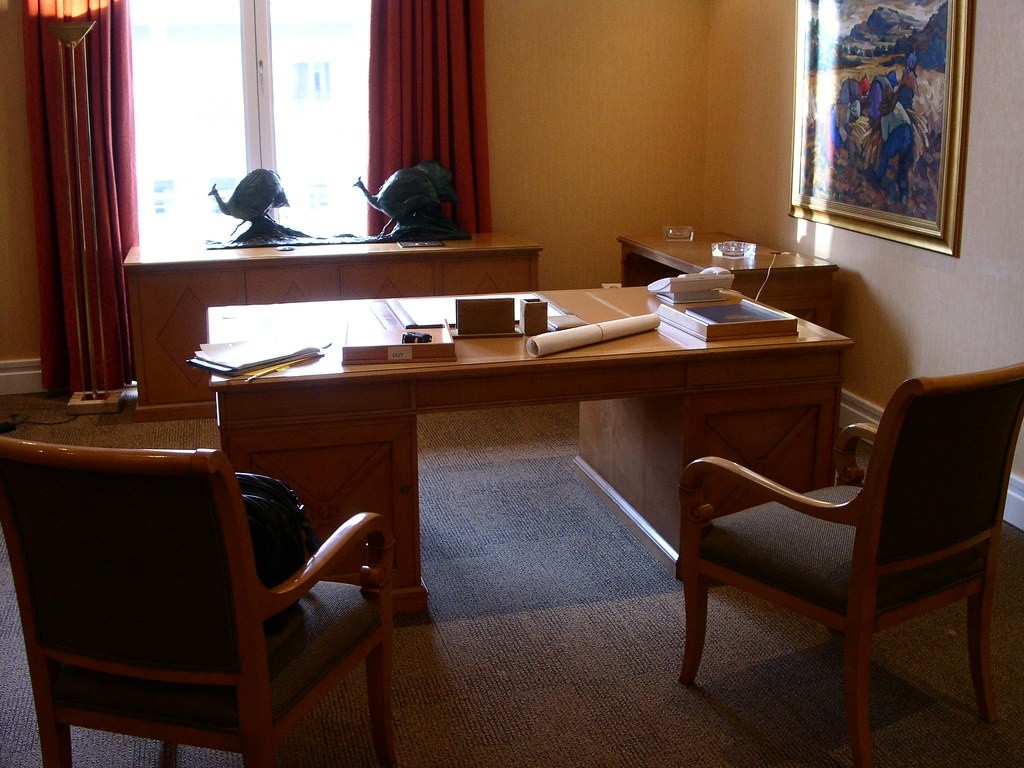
[674,358,1024,768]
[0,435,400,768]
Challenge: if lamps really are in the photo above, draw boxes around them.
[44,20,127,416]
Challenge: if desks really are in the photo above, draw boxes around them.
[205,284,852,619]
[615,230,839,333]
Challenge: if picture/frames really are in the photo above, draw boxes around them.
[789,0,977,259]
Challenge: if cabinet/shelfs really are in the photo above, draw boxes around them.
[122,232,544,421]
